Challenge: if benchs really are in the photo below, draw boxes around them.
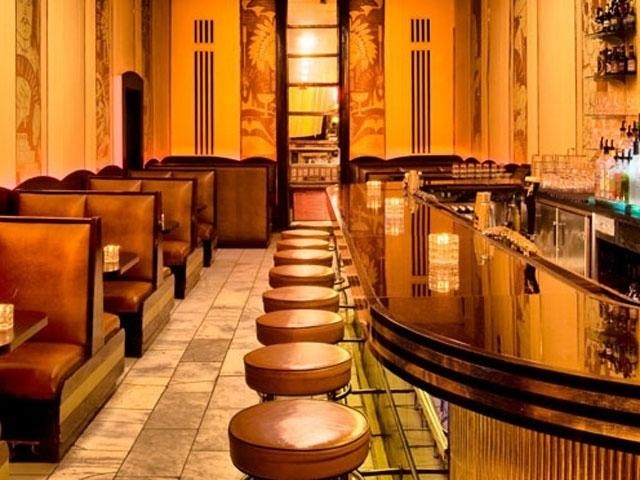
[0,215,126,462]
[88,162,273,301]
[11,187,176,359]
[352,155,495,183]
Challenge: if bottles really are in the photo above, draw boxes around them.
[474,188,537,244]
[594,120,640,219]
[405,169,424,195]
[594,2,637,76]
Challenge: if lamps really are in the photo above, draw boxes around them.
[366,181,460,292]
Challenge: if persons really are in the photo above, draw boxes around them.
[329,123,337,134]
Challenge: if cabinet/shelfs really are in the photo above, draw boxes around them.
[533,198,591,282]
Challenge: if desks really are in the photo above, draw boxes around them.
[2,306,50,358]
[102,250,140,277]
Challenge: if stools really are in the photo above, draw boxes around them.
[227,399,372,480]
[255,310,345,344]
[281,229,332,240]
[243,341,352,403]
[262,285,340,312]
[275,237,329,253]
[272,247,334,267]
[267,262,334,287]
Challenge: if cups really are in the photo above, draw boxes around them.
[529,151,595,202]
[429,233,460,265]
[366,181,382,197]
[366,196,382,208]
[103,243,121,263]
[451,162,511,181]
[384,217,405,236]
[0,306,17,331]
[383,196,404,218]
[429,265,460,293]
